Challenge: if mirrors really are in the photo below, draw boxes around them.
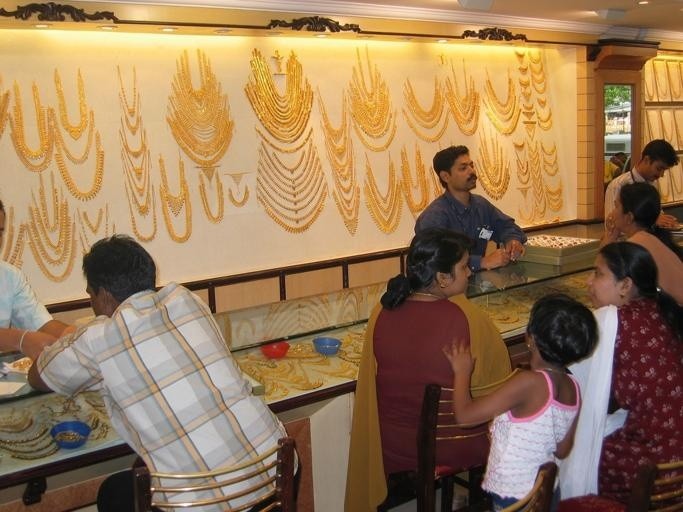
[604,84,632,191]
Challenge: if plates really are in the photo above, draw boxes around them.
[0,382,26,396]
[4,355,30,374]
[659,225,683,240]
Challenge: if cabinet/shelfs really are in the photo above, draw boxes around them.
[0,232,596,502]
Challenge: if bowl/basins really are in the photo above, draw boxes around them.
[312,337,341,355]
[260,341,290,359]
[50,421,90,449]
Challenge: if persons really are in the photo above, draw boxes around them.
[0,201,78,362]
[553,241,683,512]
[445,294,600,512]
[373,226,515,512]
[414,145,528,272]
[28,233,298,512]
[597,140,683,306]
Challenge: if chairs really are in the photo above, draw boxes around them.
[376,363,531,511]
[133,437,295,512]
